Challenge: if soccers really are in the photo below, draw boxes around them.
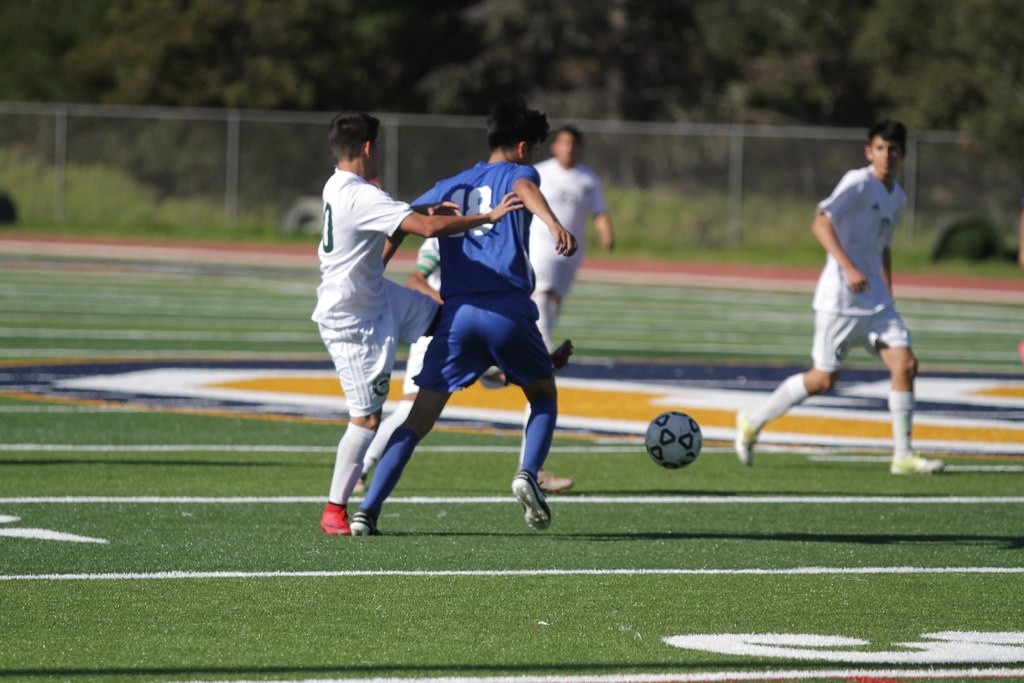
[645,410,703,470]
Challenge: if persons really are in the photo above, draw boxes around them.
[311,112,523,536]
[352,237,573,493]
[348,109,578,537]
[734,120,945,475]
[527,124,614,354]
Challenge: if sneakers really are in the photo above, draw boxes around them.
[889,449,944,475]
[735,410,767,467]
[321,502,351,535]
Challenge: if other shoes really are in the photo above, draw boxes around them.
[551,340,573,373]
[352,478,366,492]
[349,508,382,537]
[536,471,574,493]
[512,470,551,531]
